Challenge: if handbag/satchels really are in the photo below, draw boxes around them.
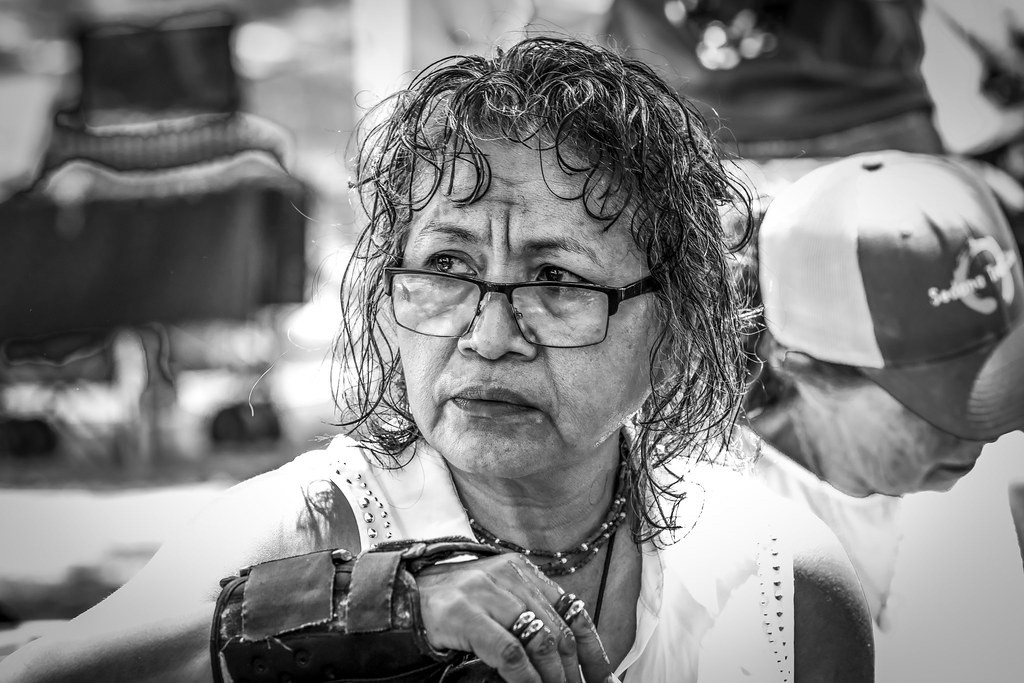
[72,7,240,116]
[977,48,1024,114]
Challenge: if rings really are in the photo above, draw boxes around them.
[556,590,585,622]
[514,608,545,644]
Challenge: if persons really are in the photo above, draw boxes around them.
[2,34,1024,683]
[602,1,1024,683]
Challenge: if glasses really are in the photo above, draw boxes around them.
[382,251,684,349]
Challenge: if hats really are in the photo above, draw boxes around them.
[757,146,1024,441]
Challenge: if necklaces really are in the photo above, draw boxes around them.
[447,426,635,581]
